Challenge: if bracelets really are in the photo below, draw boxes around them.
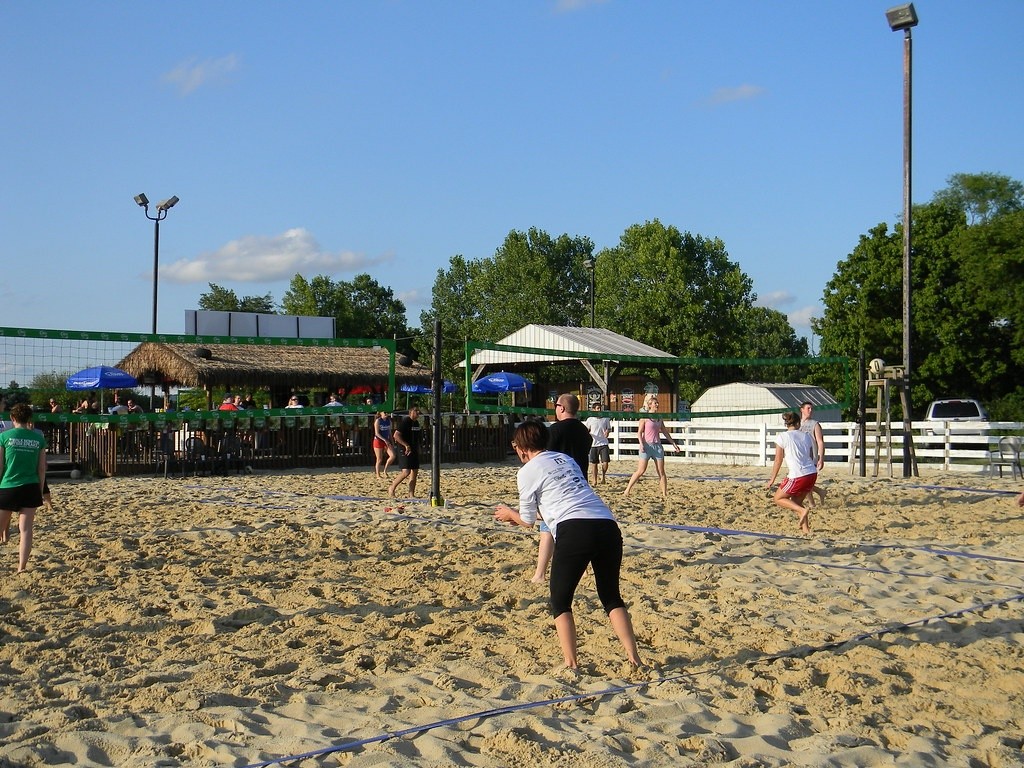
[405,444,410,448]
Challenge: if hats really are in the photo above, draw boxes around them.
[223,393,233,400]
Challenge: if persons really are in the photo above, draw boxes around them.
[321,394,346,454]
[162,403,174,414]
[494,393,644,671]
[364,397,377,408]
[72,396,146,455]
[624,397,680,501]
[387,403,424,502]
[373,406,397,480]
[764,409,818,533]
[797,402,827,509]
[281,395,305,409]
[0,403,54,574]
[48,398,62,453]
[215,391,259,458]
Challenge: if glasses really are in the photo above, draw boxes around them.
[384,411,389,414]
[554,403,562,408]
[291,398,298,401]
[51,402,56,404]
[511,441,518,450]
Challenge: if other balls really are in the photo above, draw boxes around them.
[869,358,886,374]
[70,469,81,479]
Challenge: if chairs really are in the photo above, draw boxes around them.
[989,437,1024,480]
[119,429,280,480]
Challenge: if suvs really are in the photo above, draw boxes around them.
[921,397,989,458]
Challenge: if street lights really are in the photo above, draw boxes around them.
[133,192,179,411]
[583,258,596,328]
[885,3,918,479]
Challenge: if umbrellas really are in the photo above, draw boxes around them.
[65,365,138,391]
[470,371,532,394]
[399,376,457,393]
